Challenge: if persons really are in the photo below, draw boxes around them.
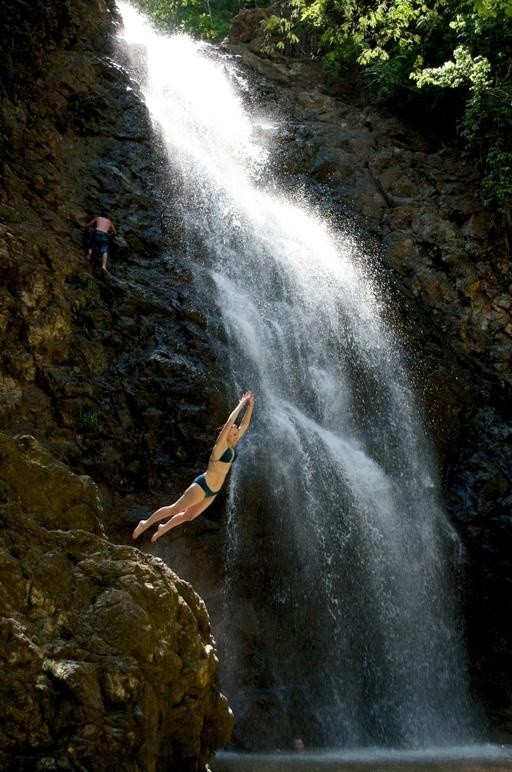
[132,391,254,542]
[293,738,305,753]
[87,213,117,273]
